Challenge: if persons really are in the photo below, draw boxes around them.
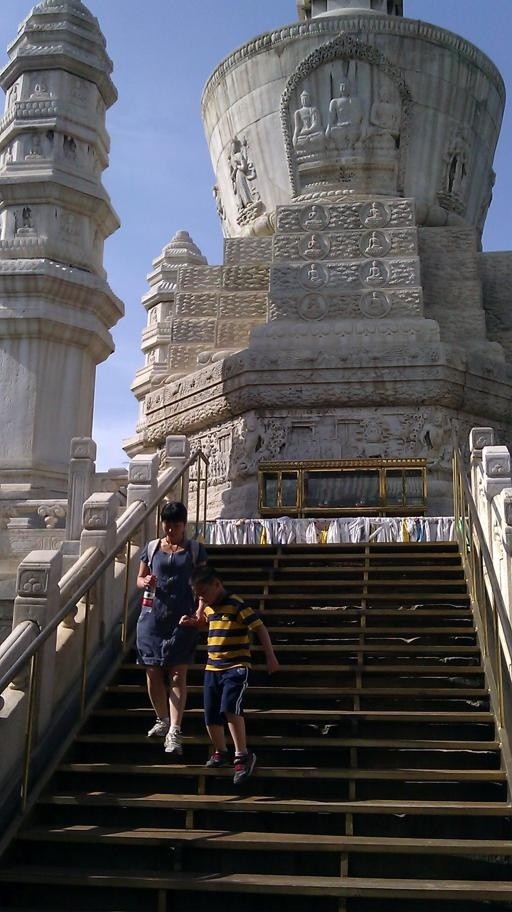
[364,202,384,316]
[176,563,281,785]
[293,78,400,149]
[305,206,323,317]
[136,497,208,753]
[228,136,253,214]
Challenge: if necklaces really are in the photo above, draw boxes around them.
[164,537,184,552]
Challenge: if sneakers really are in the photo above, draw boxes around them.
[206,744,232,769]
[162,725,185,757]
[147,715,171,738]
[232,750,257,785]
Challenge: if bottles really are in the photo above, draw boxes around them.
[141,581,155,615]
